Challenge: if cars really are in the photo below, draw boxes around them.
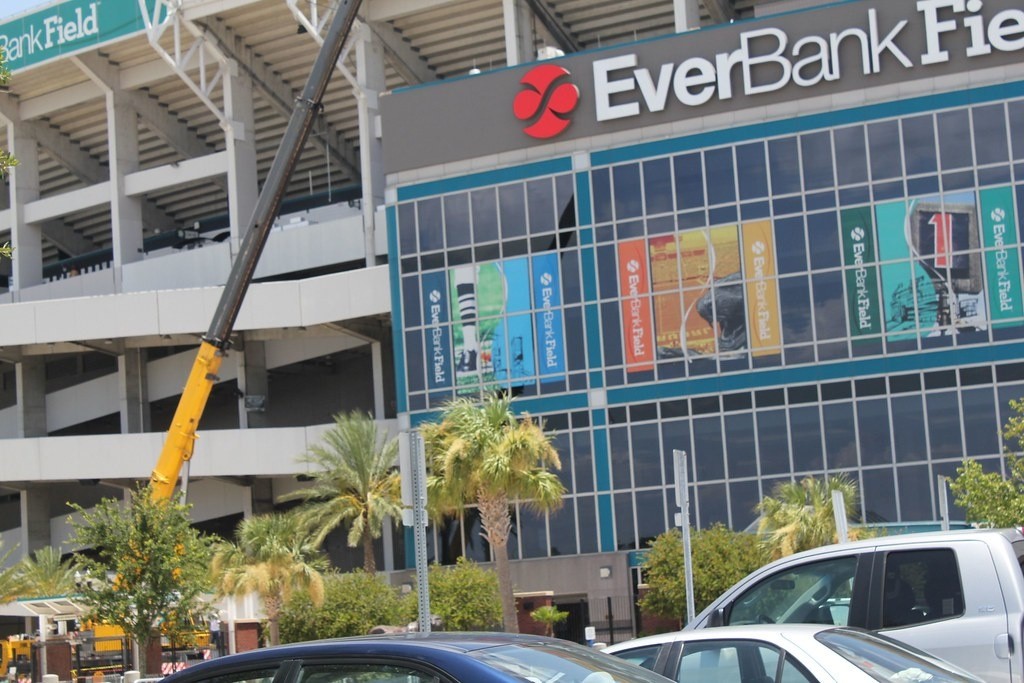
[599,621,990,681]
[157,631,681,683]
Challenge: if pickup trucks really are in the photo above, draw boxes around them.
[680,526,1023,682]
